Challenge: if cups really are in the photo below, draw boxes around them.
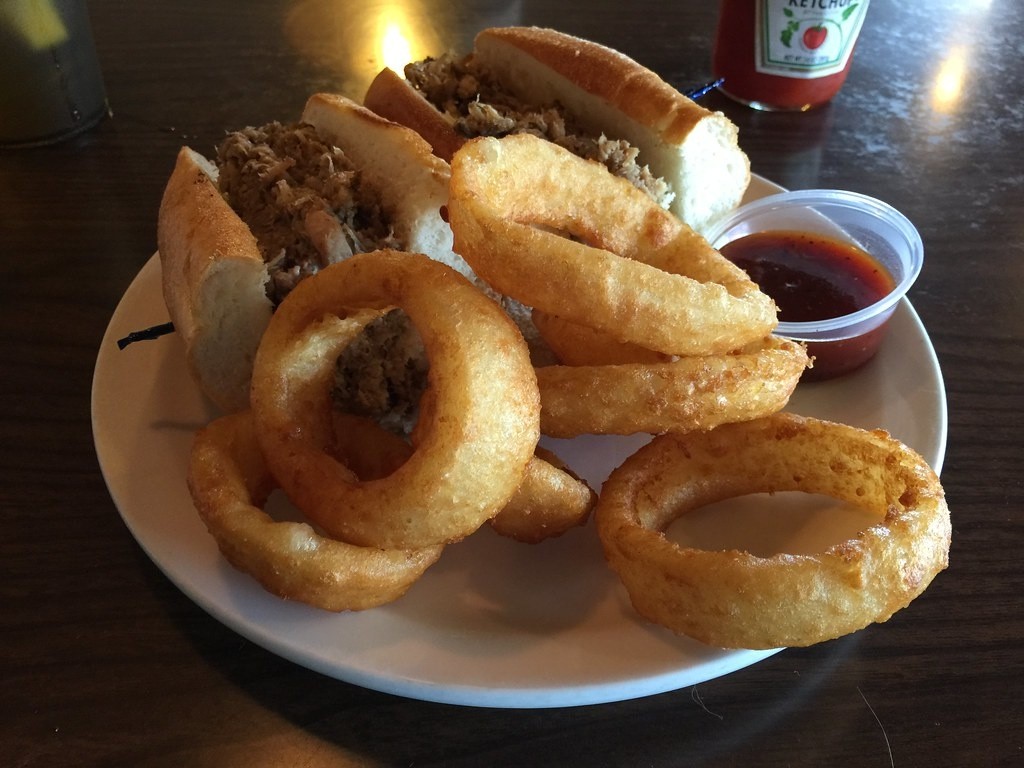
[0,1,109,149]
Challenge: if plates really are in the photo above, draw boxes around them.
[91,172,948,709]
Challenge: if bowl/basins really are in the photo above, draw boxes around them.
[703,188,924,381]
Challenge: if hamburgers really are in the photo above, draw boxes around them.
[157,93,475,410]
[361,22,753,242]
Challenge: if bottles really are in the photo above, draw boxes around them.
[711,0,870,113]
